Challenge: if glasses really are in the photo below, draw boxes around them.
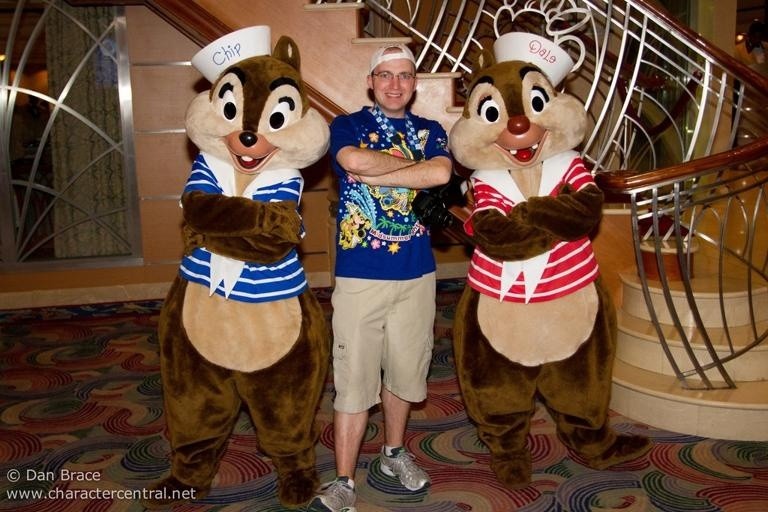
[376,70,414,82]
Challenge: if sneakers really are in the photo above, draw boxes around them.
[380,447,432,493]
[305,478,357,512]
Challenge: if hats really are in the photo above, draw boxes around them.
[369,43,416,74]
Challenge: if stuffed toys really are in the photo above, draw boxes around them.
[140,23,329,510]
[448,32,654,488]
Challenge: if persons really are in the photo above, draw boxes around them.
[9,94,53,176]
[732,19,768,150]
[308,41,454,512]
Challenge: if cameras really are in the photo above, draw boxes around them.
[411,176,469,228]
